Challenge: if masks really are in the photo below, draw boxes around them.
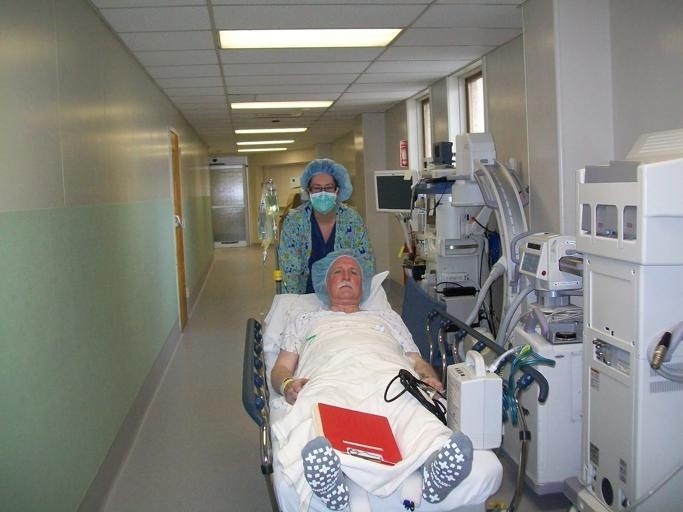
[306,186,340,216]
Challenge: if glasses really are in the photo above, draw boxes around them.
[308,182,338,192]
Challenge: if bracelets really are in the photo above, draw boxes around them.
[280,377,295,394]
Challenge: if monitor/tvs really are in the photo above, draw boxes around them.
[374,171,416,212]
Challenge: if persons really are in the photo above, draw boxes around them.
[270,248,476,511]
[276,158,377,294]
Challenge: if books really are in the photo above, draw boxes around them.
[311,402,403,466]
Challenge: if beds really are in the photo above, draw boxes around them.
[243,276,550,511]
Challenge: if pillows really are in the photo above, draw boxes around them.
[271,278,391,328]
